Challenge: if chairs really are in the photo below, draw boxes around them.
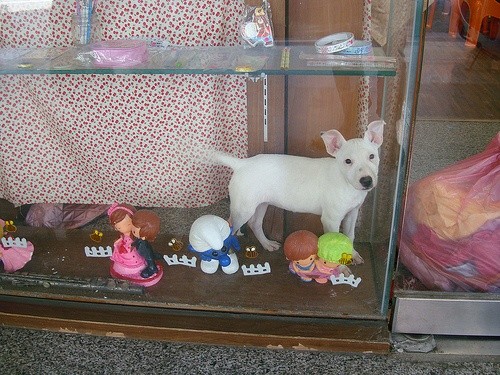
[463,15,500,74]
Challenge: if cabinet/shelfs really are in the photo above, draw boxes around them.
[0,1,425,354]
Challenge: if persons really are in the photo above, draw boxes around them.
[308,1,406,172]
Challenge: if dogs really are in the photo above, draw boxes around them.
[208,120,384,278]
